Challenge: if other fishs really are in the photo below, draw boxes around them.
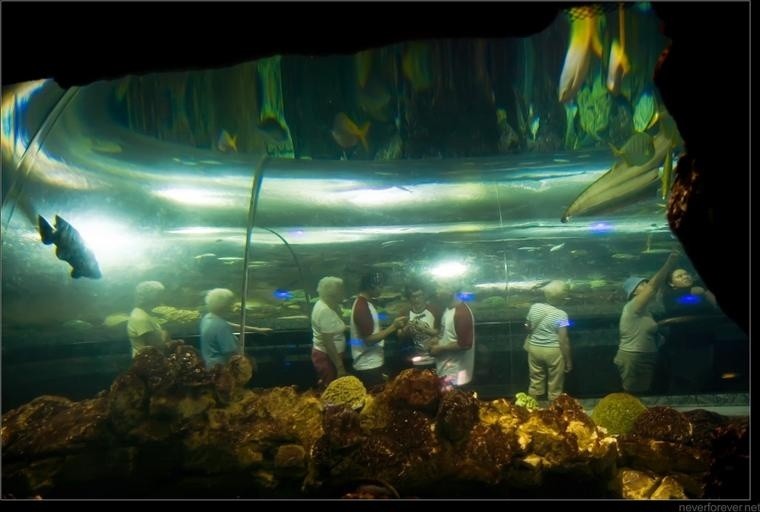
[60,33,556,185]
[105,238,727,372]
[38,216,103,281]
[556,3,682,261]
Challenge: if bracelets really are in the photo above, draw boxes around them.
[702,289,707,298]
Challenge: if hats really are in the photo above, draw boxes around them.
[622,275,649,300]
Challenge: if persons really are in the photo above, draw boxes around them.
[349,267,407,391]
[395,281,442,376]
[655,266,722,401]
[611,248,681,399]
[424,270,476,395]
[126,280,185,358]
[309,276,348,385]
[524,279,574,406]
[198,286,245,372]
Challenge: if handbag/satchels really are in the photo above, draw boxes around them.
[523,334,530,352]
